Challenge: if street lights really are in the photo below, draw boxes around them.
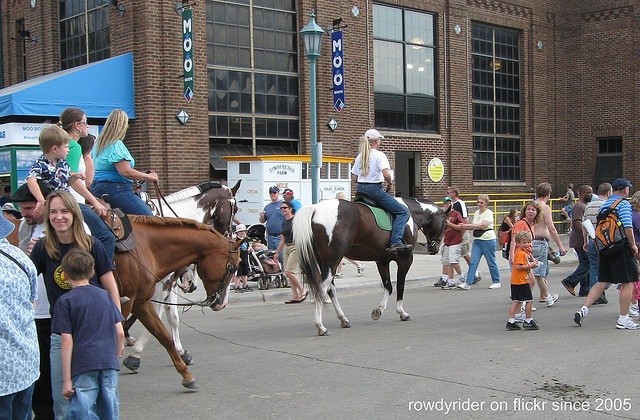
[299,13,325,204]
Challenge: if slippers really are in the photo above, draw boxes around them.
[285,290,308,304]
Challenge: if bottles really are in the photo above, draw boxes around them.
[533,261,544,268]
[555,251,561,257]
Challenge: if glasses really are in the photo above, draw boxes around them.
[478,201,484,204]
[280,206,289,209]
[447,192,454,194]
[270,192,276,194]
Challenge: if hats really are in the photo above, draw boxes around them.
[8,183,52,202]
[442,195,454,203]
[365,128,384,140]
[235,223,247,233]
[280,189,293,196]
[612,178,633,190]
[2,203,16,211]
[269,186,280,192]
[0,214,15,240]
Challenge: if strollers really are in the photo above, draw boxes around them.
[245,223,287,289]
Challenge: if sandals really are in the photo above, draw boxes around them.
[298,291,308,302]
[285,298,298,304]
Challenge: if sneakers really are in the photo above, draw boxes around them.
[561,279,576,296]
[545,294,559,307]
[234,286,245,293]
[574,310,584,326]
[442,281,456,290]
[489,282,502,289]
[243,285,253,292]
[335,272,343,278]
[506,322,521,330]
[386,243,412,260]
[434,279,448,287]
[458,282,470,290]
[616,318,639,329]
[473,274,482,283]
[515,308,526,322]
[357,264,366,276]
[524,322,539,331]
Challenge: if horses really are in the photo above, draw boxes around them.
[0,210,248,390]
[122,179,244,374]
[292,196,453,336]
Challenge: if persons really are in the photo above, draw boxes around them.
[441,196,466,290]
[559,183,575,234]
[11,181,52,420]
[629,190,640,316]
[434,186,482,286]
[498,209,522,248]
[26,125,87,218]
[505,230,539,330]
[560,185,593,298]
[0,211,41,419]
[349,129,414,255]
[50,247,125,420]
[522,182,566,312]
[56,108,131,304]
[248,237,278,268]
[0,185,11,205]
[89,110,155,216]
[234,224,253,294]
[229,215,242,290]
[573,178,640,331]
[333,192,366,279]
[29,190,122,419]
[508,201,544,323]
[274,204,308,302]
[77,134,94,189]
[280,188,302,214]
[581,182,613,303]
[259,186,289,273]
[3,202,22,219]
[457,194,502,290]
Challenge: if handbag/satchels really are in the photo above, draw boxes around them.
[500,223,511,245]
[473,229,494,237]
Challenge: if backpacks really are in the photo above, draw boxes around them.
[595,196,627,256]
